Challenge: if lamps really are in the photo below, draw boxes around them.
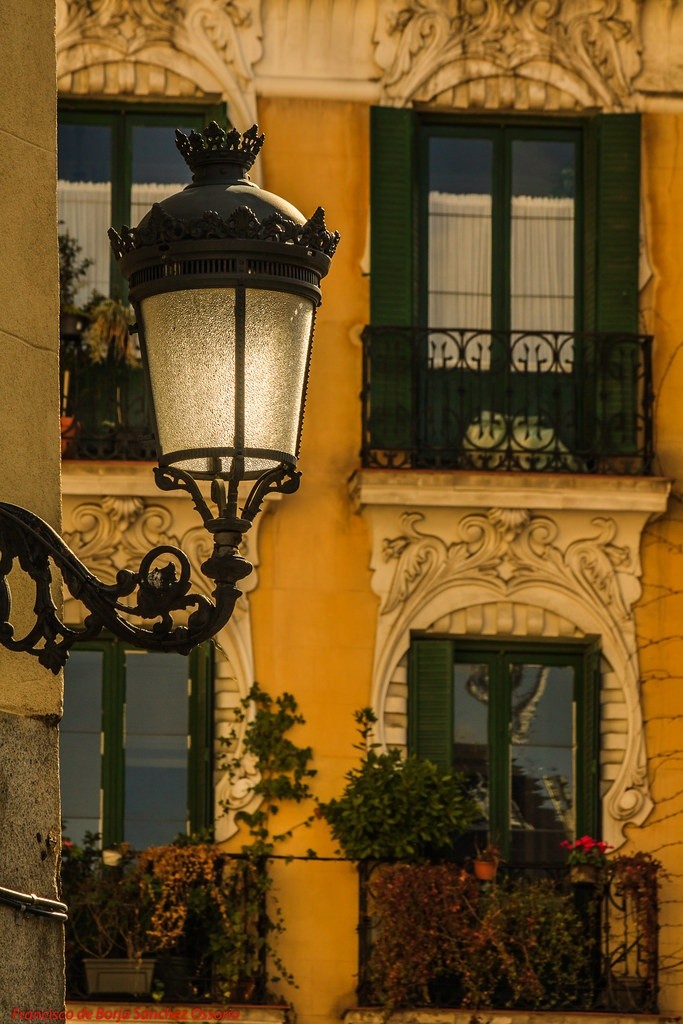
[1,121,341,678]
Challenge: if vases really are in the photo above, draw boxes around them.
[474,861,498,879]
[82,957,155,996]
[570,864,598,882]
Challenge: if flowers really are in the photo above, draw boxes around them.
[61,819,164,960]
[560,836,613,868]
[476,844,500,861]
